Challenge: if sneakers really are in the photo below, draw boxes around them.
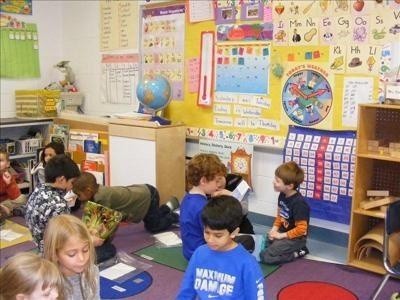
[296,246,309,257]
[168,196,181,217]
[13,208,26,216]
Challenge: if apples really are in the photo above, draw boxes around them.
[353,0,364,11]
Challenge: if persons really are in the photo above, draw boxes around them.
[259,161,311,264]
[0,251,64,300]
[43,214,100,300]
[0,142,255,265]
[174,195,267,300]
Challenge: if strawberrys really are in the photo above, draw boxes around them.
[275,2,284,15]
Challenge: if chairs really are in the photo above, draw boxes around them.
[369,200,400,300]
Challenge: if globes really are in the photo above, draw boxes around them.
[136,71,173,126]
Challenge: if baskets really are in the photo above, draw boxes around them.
[14,139,43,154]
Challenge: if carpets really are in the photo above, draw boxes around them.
[0,213,400,300]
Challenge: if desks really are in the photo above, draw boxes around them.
[53,115,109,186]
[109,121,186,210]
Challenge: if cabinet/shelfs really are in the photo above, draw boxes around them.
[346,103,400,277]
[0,118,52,189]
